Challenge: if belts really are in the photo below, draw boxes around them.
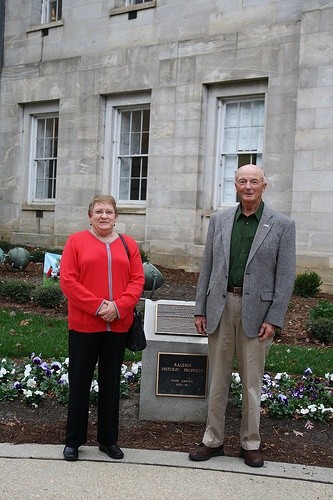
[227,286,242,296]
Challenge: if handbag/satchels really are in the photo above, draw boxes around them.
[125,312,147,351]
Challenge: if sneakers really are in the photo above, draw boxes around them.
[189,442,225,461]
[239,446,264,467]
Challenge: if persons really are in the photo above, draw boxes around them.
[59,194,146,461]
[188,163,297,468]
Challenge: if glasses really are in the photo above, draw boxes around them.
[92,210,116,215]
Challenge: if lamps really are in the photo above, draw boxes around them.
[137,262,165,300]
[0,247,33,272]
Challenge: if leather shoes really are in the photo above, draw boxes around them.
[99,443,124,459]
[63,446,78,460]
